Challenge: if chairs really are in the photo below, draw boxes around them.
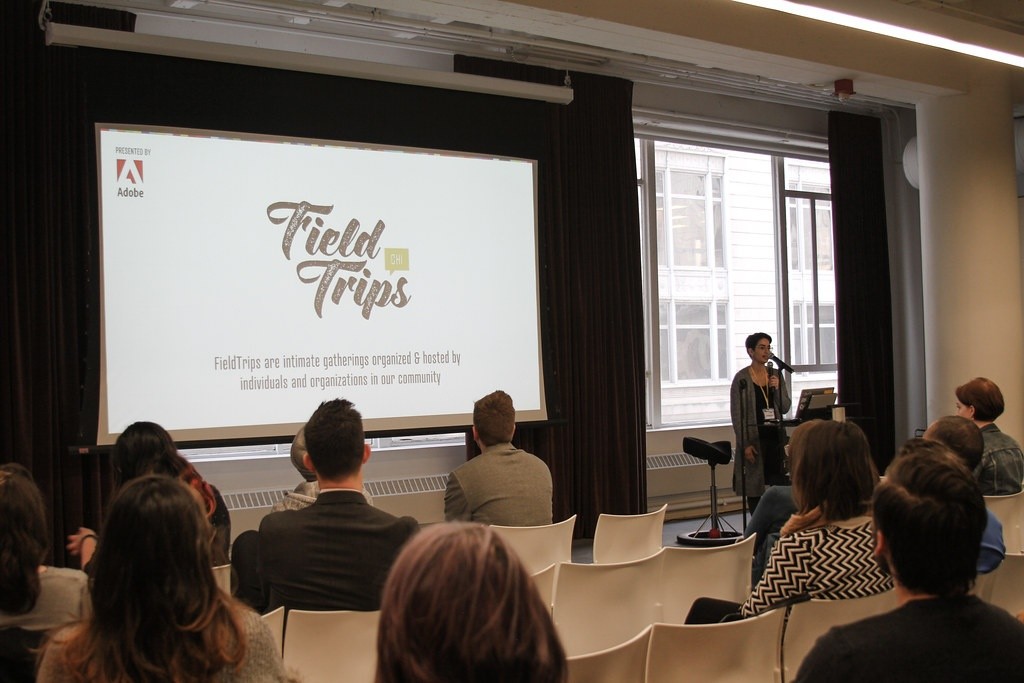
[213,477,1024,683]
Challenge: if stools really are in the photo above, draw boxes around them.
[677,436,743,547]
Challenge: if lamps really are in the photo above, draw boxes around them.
[39,0,574,106]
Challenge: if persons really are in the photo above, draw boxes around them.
[684,421,894,649]
[735,419,823,593]
[794,436,1024,683]
[270,428,373,513]
[923,415,1005,574]
[68,420,231,575]
[445,392,553,526]
[376,519,569,683]
[955,376,1024,495]
[0,463,307,683]
[730,333,791,516]
[231,395,420,658]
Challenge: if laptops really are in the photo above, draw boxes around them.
[783,386,837,422]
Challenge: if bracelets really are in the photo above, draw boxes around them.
[78,535,97,548]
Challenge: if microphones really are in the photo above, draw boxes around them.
[769,353,794,374]
[767,361,775,393]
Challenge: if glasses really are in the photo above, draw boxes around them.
[915,429,948,447]
[754,345,773,352]
[784,445,791,456]
